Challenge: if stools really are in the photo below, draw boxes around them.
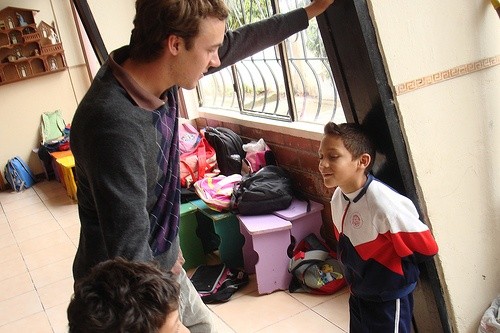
[273,197,327,254]
[236,214,293,295]
[48,150,78,202]
[180,202,207,271]
[190,198,246,270]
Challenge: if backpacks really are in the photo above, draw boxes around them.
[204,126,246,177]
[190,263,251,305]
[230,164,292,215]
[4,157,36,192]
[288,233,347,294]
[193,174,244,212]
[241,150,277,178]
[40,109,66,145]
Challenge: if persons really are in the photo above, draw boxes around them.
[67,257,192,332]
[318,122,439,333]
[69,0,334,332]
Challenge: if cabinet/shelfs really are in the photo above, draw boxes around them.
[0,6,67,86]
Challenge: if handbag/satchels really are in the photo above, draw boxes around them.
[178,137,222,189]
